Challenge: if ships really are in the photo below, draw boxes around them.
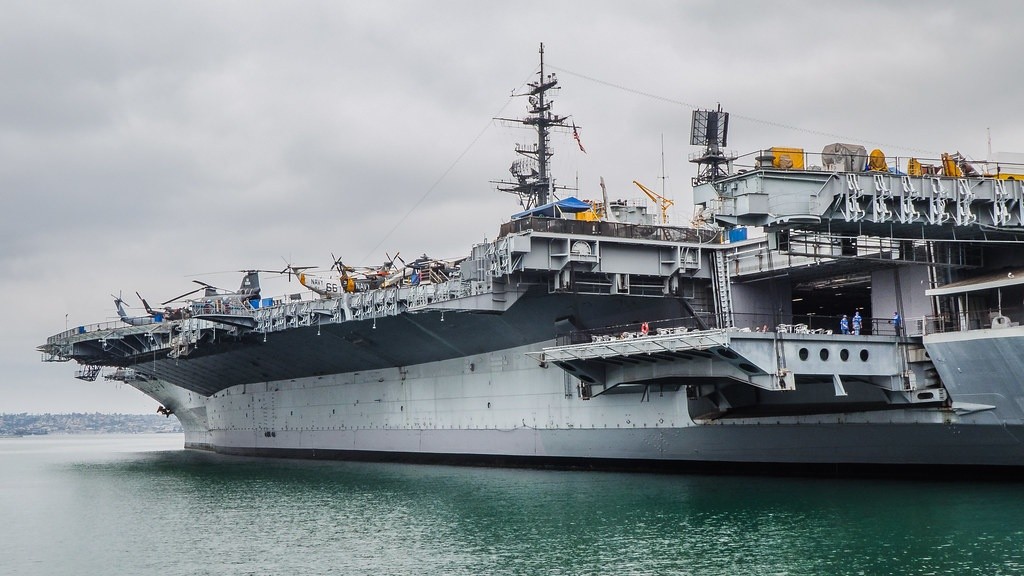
[33,44,1023,469]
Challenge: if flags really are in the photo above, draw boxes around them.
[573,122,587,154]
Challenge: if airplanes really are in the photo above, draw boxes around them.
[110,252,460,327]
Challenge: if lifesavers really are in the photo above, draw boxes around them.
[642,322,648,334]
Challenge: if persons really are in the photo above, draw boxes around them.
[840,315,849,335]
[893,312,901,337]
[852,312,862,335]
